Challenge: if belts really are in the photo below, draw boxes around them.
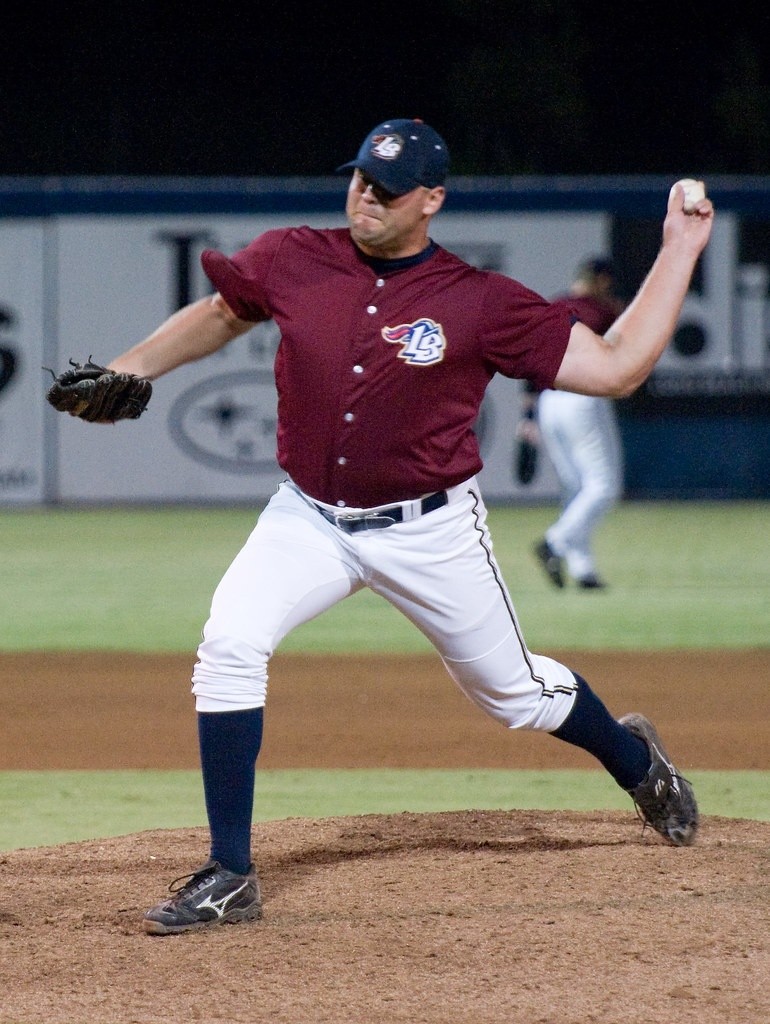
[312,490,449,533]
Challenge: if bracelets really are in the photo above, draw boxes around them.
[519,404,537,421]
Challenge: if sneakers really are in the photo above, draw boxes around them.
[141,860,264,934]
[616,712,699,845]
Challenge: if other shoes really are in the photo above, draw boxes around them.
[576,574,606,594]
[537,543,564,588]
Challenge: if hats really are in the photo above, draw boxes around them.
[338,118,451,196]
[585,255,627,280]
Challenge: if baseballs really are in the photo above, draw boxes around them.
[669,178,706,215]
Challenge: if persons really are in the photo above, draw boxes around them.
[73,109,717,935]
[515,252,623,591]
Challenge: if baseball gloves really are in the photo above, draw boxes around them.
[47,363,153,425]
